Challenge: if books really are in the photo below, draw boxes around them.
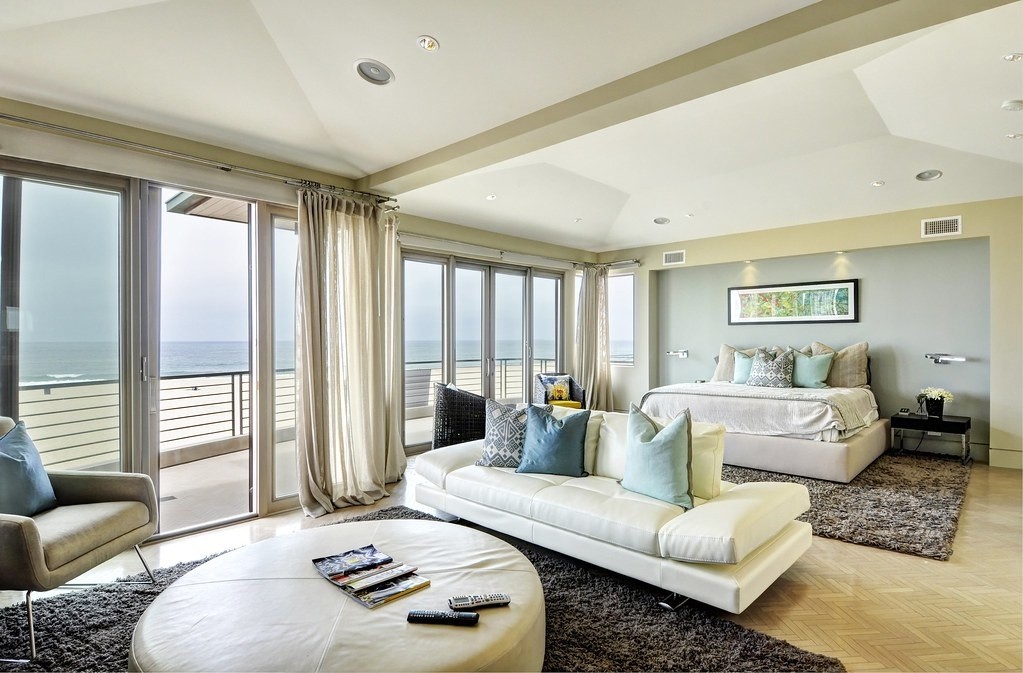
[312,544,430,611]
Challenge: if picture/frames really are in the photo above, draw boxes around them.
[727,279,859,326]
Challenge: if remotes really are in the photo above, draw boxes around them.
[407,611,479,625]
[448,593,511,611]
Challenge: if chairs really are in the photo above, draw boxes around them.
[1,416,159,664]
[432,382,486,449]
[534,373,586,409]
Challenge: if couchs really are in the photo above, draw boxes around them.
[414,403,813,616]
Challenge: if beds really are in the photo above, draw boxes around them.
[638,356,895,484]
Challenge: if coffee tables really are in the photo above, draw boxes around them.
[128,519,547,673]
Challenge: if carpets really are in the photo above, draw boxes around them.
[719,448,974,563]
[1,506,848,672]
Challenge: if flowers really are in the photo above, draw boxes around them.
[915,386,954,403]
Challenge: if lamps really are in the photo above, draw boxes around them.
[924,353,966,364]
[667,350,688,358]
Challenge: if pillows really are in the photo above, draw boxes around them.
[541,374,570,401]
[474,398,554,467]
[710,342,871,390]
[0,420,57,516]
[515,403,590,477]
[616,402,695,508]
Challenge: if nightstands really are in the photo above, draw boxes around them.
[890,413,971,465]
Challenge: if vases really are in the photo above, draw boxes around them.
[924,398,944,418]
